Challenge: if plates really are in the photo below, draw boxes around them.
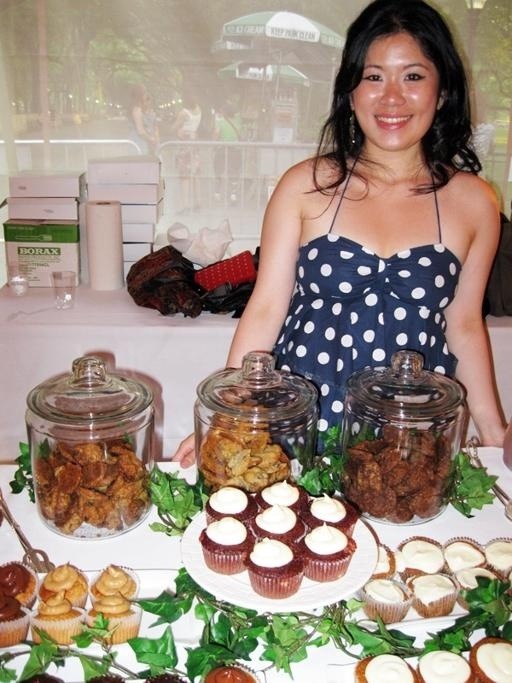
[179,510,379,611]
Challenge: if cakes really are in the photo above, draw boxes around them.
[203,478,358,597]
[358,538,512,623]
[471,637,512,683]
[201,662,257,683]
[416,650,472,683]
[357,653,416,683]
[0,559,140,650]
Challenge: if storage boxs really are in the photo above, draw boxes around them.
[2,217,81,288]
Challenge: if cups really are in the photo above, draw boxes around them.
[0,563,143,649]
[356,536,512,621]
[21,662,262,682]
[355,638,512,683]
[50,269,77,308]
[205,480,362,596]
[8,261,34,295]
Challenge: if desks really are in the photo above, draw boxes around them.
[2,275,511,440]
[2,444,511,682]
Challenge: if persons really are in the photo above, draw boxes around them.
[125,83,161,155]
[463,91,496,180]
[172,1,508,469]
[169,92,202,218]
[211,104,246,208]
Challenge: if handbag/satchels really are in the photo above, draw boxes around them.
[195,250,257,289]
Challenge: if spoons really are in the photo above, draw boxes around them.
[0,493,54,573]
[472,454,512,521]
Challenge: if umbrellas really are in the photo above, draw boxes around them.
[203,9,345,146]
